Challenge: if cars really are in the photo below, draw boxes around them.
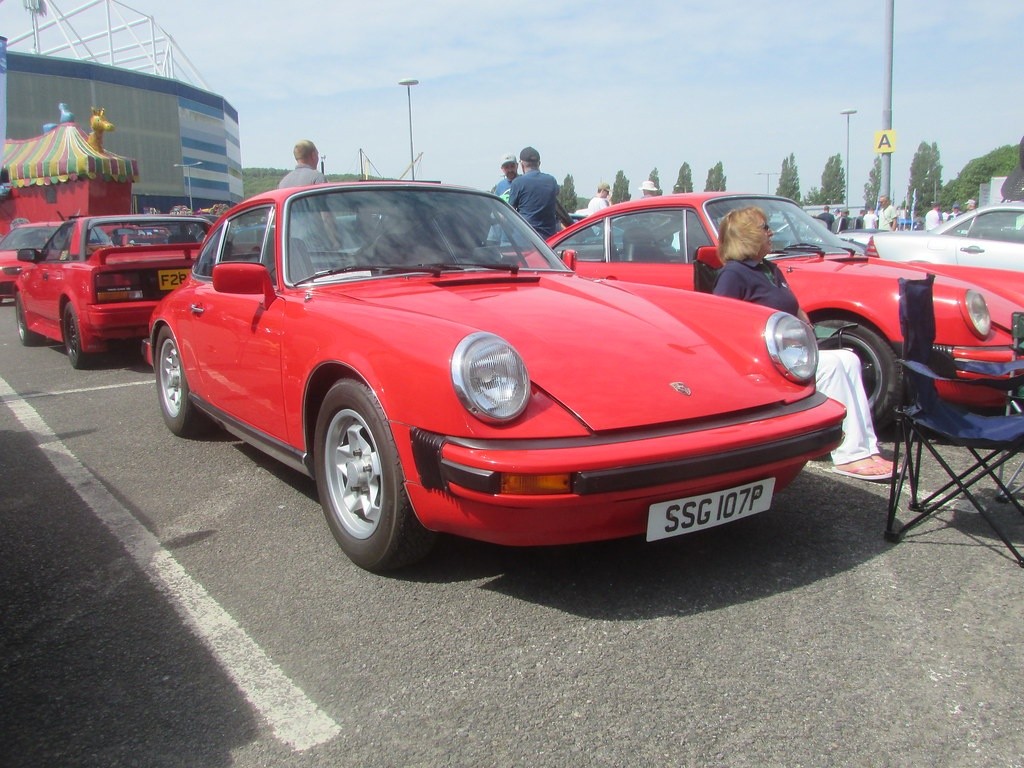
[837,202,1024,273]
[500,192,1023,435]
[0,213,217,370]
[141,181,848,575]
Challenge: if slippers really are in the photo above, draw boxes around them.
[875,459,901,473]
[832,463,892,479]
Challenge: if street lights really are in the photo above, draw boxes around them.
[173,162,203,213]
[838,108,858,211]
[398,78,419,182]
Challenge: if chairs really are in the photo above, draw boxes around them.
[623,225,667,262]
[884,272,1024,569]
[996,312,1024,502]
[694,260,857,350]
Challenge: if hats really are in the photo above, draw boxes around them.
[520,147,540,162]
[638,181,658,191]
[500,154,516,167]
[599,183,611,192]
[965,200,976,205]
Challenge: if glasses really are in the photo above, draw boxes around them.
[763,225,768,231]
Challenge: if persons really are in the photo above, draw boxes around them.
[639,181,657,196]
[817,195,898,231]
[588,183,610,217]
[713,207,904,479]
[490,147,559,247]
[914,197,976,231]
[279,140,342,252]
